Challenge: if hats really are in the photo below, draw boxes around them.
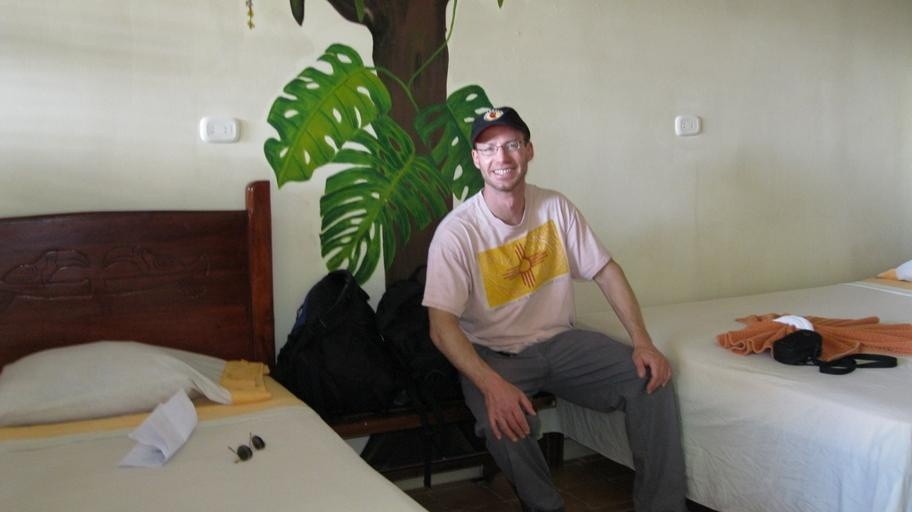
[469,108,534,149]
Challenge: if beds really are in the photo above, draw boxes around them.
[557,259,912,512]
[0,179,434,512]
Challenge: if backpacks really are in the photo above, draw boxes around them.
[273,266,397,416]
[375,264,477,439]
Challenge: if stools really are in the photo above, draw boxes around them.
[326,377,555,489]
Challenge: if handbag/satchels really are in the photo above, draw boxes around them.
[771,328,824,365]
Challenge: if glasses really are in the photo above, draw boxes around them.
[473,140,527,155]
[226,430,269,465]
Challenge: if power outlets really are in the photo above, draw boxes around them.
[675,115,702,137]
[200,116,241,144]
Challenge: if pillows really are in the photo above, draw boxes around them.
[0,339,269,426]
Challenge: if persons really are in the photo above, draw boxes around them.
[416,104,688,511]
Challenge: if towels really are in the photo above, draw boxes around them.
[716,304,912,363]
